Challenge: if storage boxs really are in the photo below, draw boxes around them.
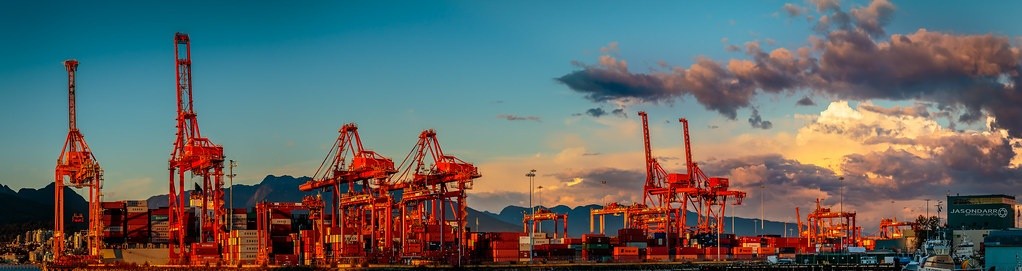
[226,209,367,263]
[101,198,172,243]
[409,221,875,263]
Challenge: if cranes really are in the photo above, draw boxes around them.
[373,129,482,265]
[169,32,226,263]
[673,117,743,244]
[50,60,104,257]
[633,109,695,238]
[299,121,393,262]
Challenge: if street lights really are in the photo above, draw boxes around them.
[924,199,931,241]
[760,185,766,231]
[525,172,535,238]
[529,169,537,236]
[839,177,845,254]
[538,185,544,214]
